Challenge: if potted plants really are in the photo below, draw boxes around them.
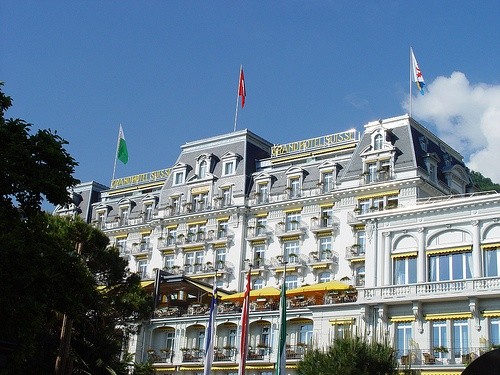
[361,169,387,175]
[285,343,307,359]
[434,345,448,352]
[423,353,437,364]
[213,345,232,351]
[369,203,397,211]
[462,353,473,365]
[179,346,201,352]
[399,354,410,365]
[82,174,361,281]
[147,348,170,355]
[247,344,267,349]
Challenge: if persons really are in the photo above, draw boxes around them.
[238,266,252,375]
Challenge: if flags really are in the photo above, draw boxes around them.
[238,65,248,110]
[116,122,130,165]
[275,266,286,375]
[409,45,426,96]
[203,269,215,375]
